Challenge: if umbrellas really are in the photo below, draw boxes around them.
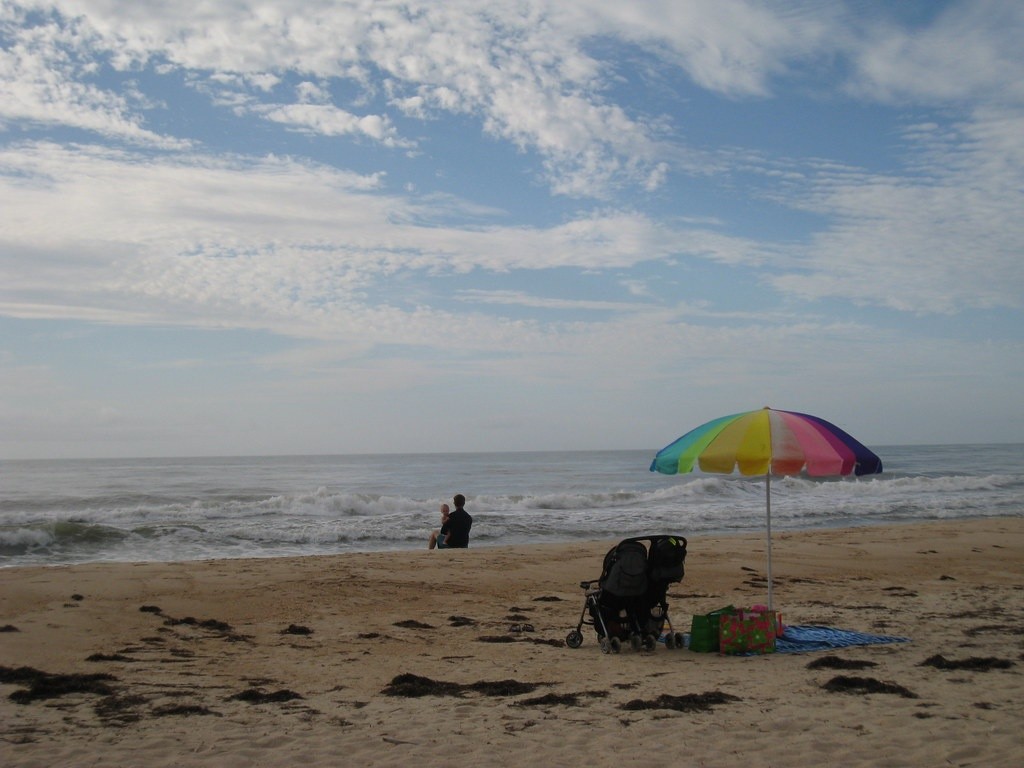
[649,406,883,609]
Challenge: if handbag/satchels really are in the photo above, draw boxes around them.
[718,606,781,657]
[689,602,735,652]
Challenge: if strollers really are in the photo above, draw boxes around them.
[565,535,688,653]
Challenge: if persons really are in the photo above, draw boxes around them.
[440,504,451,544]
[429,494,473,549]
